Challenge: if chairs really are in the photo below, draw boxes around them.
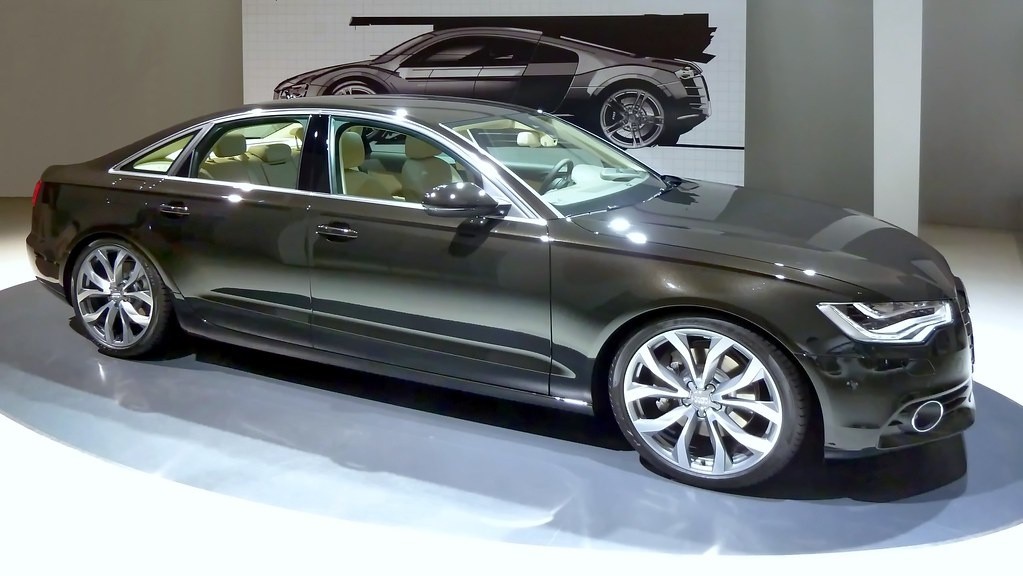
[341,132,393,201]
[401,135,462,198]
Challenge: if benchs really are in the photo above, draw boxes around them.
[203,128,307,187]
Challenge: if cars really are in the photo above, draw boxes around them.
[22,92,978,494]
